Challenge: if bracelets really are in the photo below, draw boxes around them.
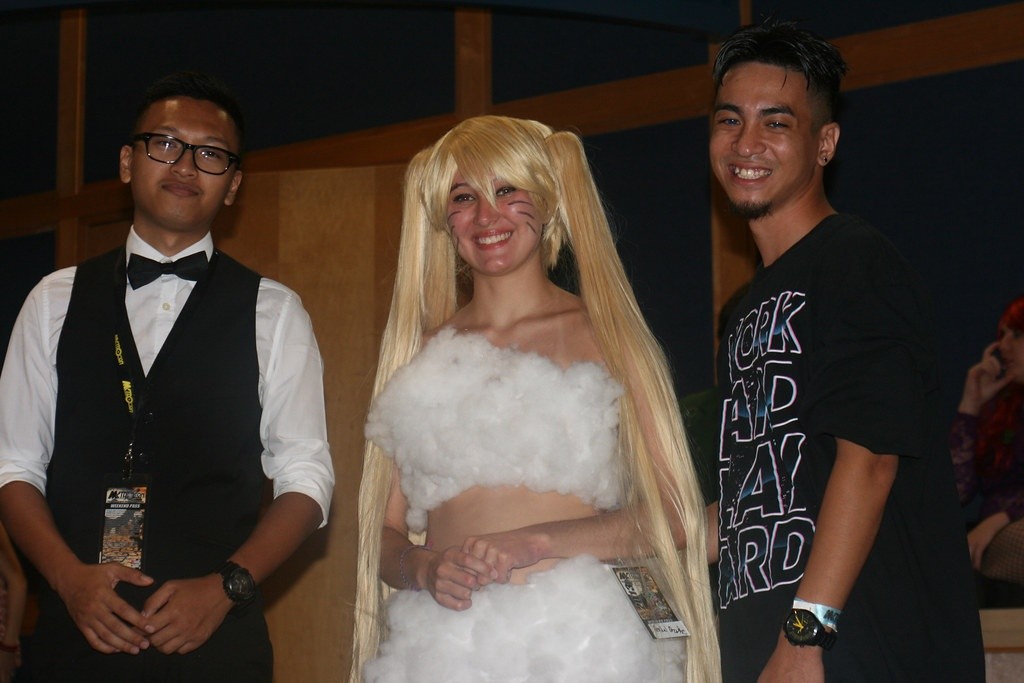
[792,597,841,633]
[0,642,18,653]
[400,540,433,592]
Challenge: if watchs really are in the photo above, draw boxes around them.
[782,608,837,653]
[213,559,257,611]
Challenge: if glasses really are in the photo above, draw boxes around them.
[132,132,241,175]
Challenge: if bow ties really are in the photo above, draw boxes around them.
[127,250,210,290]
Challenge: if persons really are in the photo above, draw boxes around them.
[949,296,1024,571]
[0,522,38,683]
[705,18,986,683]
[349,116,722,683]
[0,71,337,683]
